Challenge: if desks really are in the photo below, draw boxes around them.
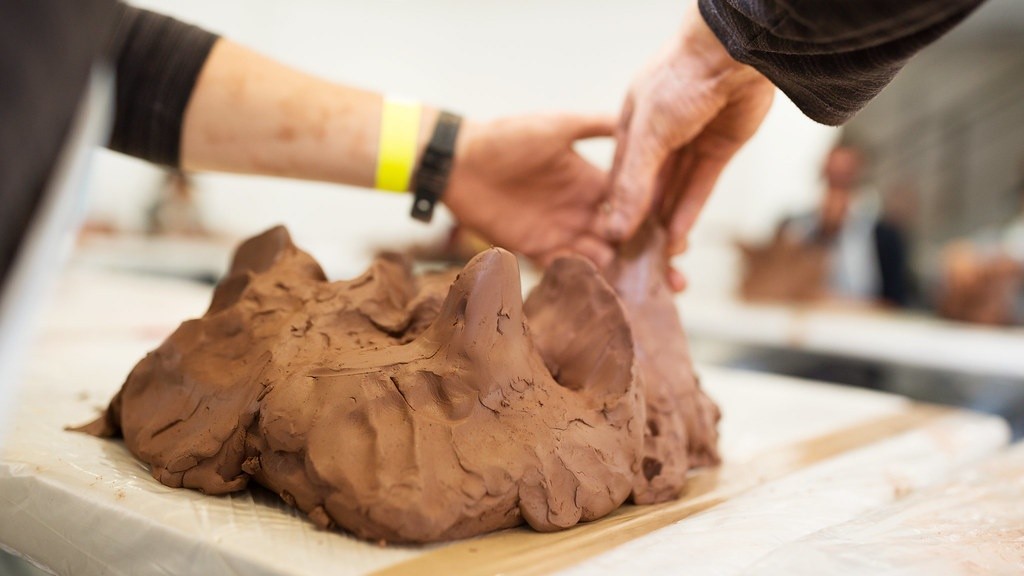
[0,268,1024,576]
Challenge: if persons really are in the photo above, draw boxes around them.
[0,0,686,308]
[592,0,986,248]
[775,141,912,307]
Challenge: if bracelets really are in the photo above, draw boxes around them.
[410,111,462,220]
[375,94,422,192]
